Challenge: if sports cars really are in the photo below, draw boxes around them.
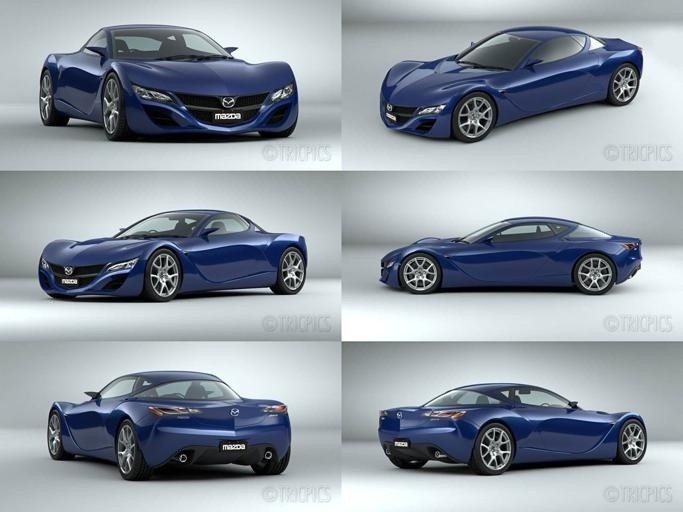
[379,383,648,476]
[38,210,307,303]
[39,25,297,141]
[47,372,291,481]
[380,216,642,295]
[380,27,643,143]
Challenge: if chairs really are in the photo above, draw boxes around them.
[211,221,227,234]
[476,395,490,405]
[115,39,132,58]
[185,384,208,400]
[158,38,179,58]
[175,222,192,236]
[535,225,542,234]
